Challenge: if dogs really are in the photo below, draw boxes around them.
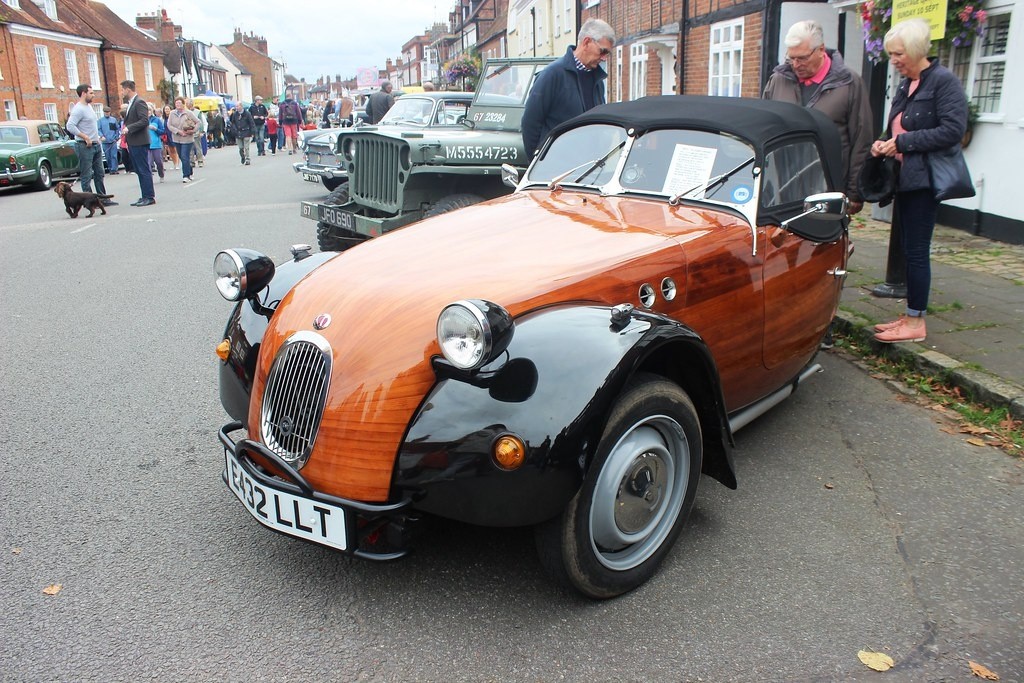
[53,180,115,218]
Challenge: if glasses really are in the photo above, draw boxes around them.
[593,39,610,55]
[784,48,816,64]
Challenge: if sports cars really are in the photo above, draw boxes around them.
[212,91,856,602]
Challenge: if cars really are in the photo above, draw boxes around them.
[0,120,80,190]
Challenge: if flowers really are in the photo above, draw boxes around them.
[856,0,987,66]
[443,53,483,92]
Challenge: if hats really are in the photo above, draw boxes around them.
[103,107,111,112]
[255,96,263,100]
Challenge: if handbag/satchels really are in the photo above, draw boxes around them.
[926,142,976,200]
[162,134,168,142]
[183,118,196,130]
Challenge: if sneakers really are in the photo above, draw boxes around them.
[873,318,906,332]
[874,319,926,343]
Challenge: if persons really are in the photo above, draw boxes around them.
[66,84,119,209]
[522,18,616,179]
[762,20,873,349]
[118,102,181,184]
[229,102,256,166]
[264,93,353,156]
[167,97,201,183]
[195,106,236,161]
[97,106,120,174]
[65,112,75,140]
[366,81,395,125]
[20,116,28,120]
[870,18,968,343]
[185,99,204,168]
[423,81,434,92]
[121,80,156,207]
[249,95,268,157]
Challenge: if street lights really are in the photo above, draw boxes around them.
[177,38,187,97]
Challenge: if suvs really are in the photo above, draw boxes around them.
[292,91,528,192]
[318,90,407,127]
[300,56,561,250]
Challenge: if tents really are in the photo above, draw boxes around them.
[183,89,309,111]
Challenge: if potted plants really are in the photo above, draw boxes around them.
[960,95,980,148]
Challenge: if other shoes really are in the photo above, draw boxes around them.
[137,198,155,206]
[160,177,165,183]
[152,158,203,171]
[241,143,300,165]
[821,334,834,349]
[102,199,118,206]
[110,172,119,175]
[189,172,194,180]
[183,177,190,183]
[130,198,142,206]
